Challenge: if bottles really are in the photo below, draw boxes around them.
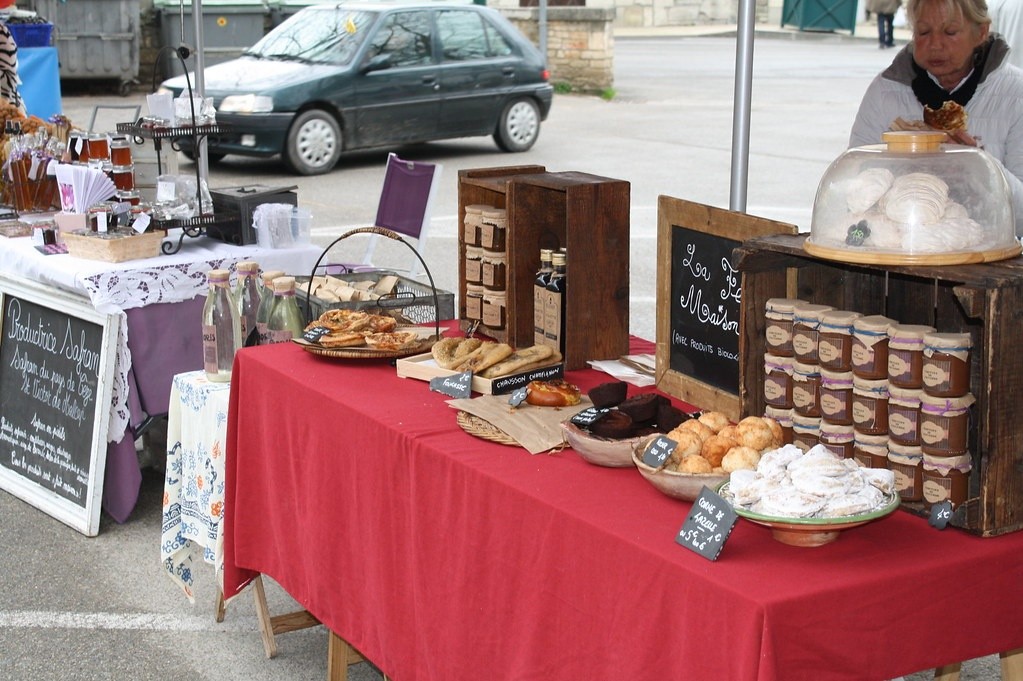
[266,276,305,343]
[1,120,67,214]
[256,271,286,344]
[532,246,566,359]
[68,130,153,233]
[201,270,242,381]
[233,262,262,348]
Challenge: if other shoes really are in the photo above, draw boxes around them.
[887,44,895,47]
[880,44,885,48]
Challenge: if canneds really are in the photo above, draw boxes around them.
[463,203,508,330]
[765,297,972,513]
[69,129,141,233]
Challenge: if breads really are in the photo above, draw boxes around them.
[524,378,581,407]
[432,337,482,369]
[587,382,782,477]
[820,167,987,253]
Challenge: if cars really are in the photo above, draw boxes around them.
[154,0,556,176]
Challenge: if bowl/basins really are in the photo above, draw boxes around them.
[252,211,312,248]
[630,436,730,503]
[589,381,628,407]
[559,407,663,468]
[713,478,900,546]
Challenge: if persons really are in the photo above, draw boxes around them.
[865,0,902,48]
[849,0,1023,183]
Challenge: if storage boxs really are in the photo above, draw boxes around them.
[252,210,314,247]
[287,269,456,329]
[209,184,299,245]
[4,22,53,47]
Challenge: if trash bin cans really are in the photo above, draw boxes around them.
[15,0,139,95]
[265,1,316,30]
[153,1,270,80]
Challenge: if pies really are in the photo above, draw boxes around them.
[922,100,968,135]
[455,342,564,377]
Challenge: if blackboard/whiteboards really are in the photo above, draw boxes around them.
[1,271,119,537]
[654,195,798,422]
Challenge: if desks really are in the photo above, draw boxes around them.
[16,46,61,121]
[1,206,328,523]
[222,348,1023,680]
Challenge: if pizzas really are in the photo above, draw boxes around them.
[306,307,418,350]
